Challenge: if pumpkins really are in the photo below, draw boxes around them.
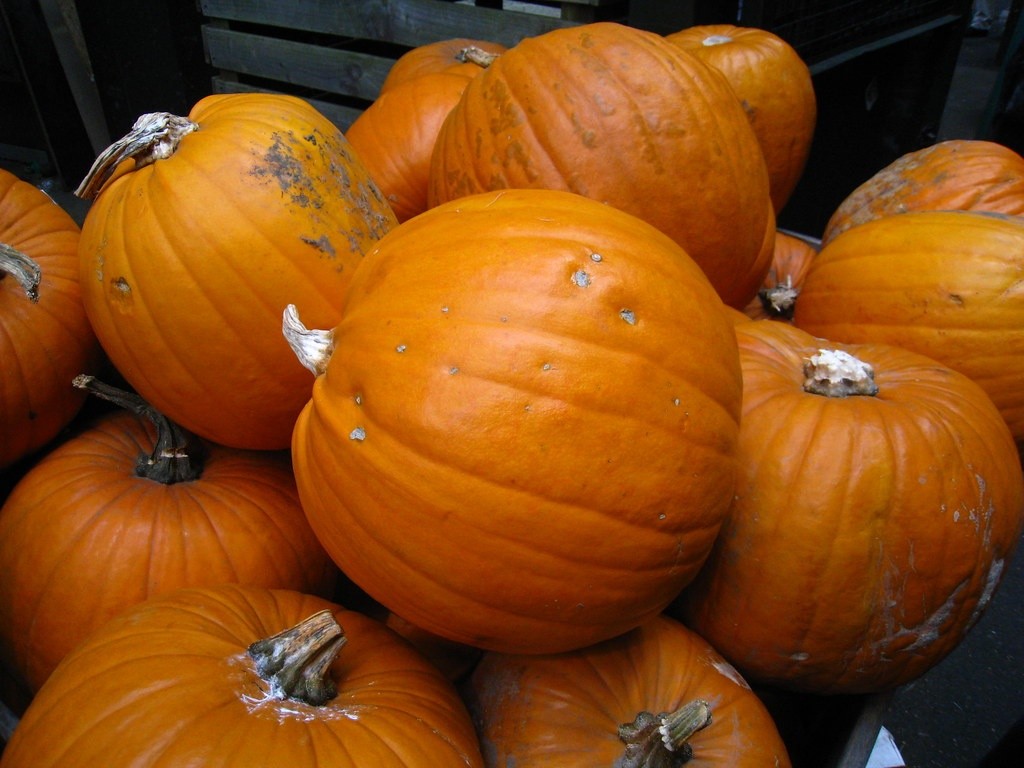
[0,23,1024,768]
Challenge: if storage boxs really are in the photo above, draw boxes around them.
[0,0,978,768]
